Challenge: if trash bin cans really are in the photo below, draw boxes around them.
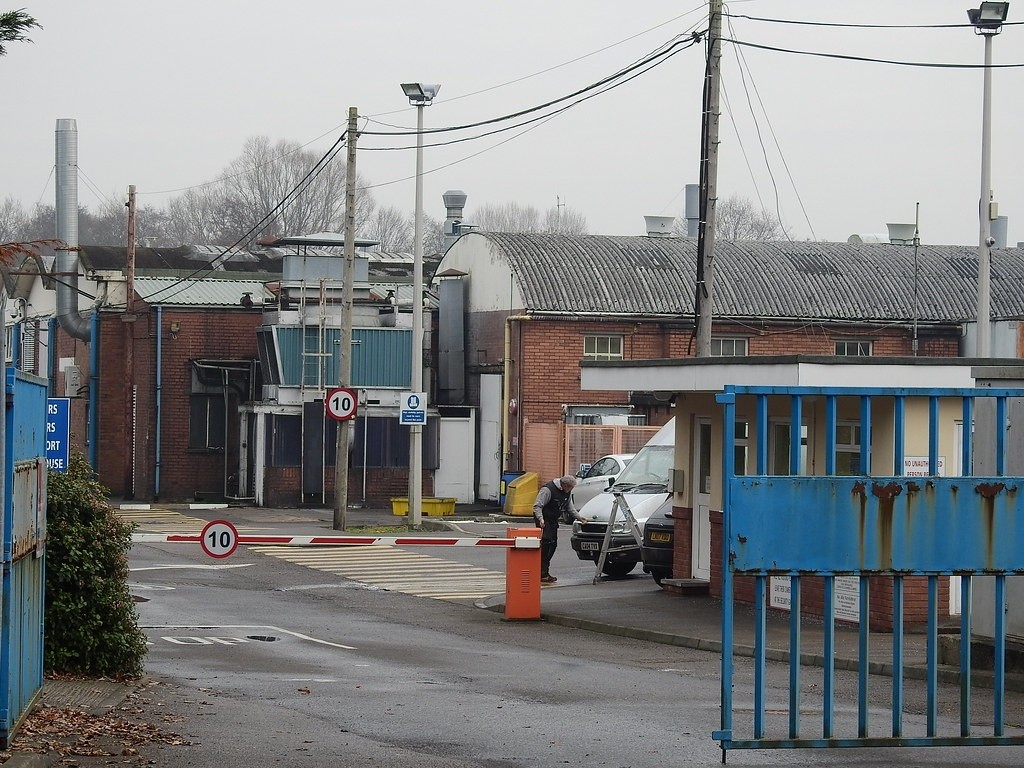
[502,472,539,517]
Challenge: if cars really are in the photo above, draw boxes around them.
[562,453,640,525]
[642,489,674,588]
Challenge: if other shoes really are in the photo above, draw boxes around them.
[541,575,557,581]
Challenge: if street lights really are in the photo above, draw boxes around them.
[400,81,443,526]
[968,2,1010,359]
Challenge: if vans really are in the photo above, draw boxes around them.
[571,414,807,577]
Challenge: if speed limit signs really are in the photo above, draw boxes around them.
[326,387,358,420]
[201,519,238,559]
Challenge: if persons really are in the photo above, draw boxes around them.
[532,475,588,583]
